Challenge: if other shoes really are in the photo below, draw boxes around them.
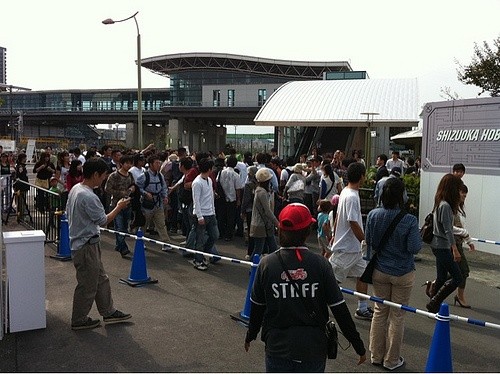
[162,245,173,251]
[246,254,250,261]
[120,248,131,256]
[426,304,432,312]
[193,260,208,270]
[224,236,232,241]
[235,232,245,238]
[384,356,405,370]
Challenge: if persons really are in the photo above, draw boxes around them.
[15,154,30,214]
[366,177,423,370]
[328,163,375,320]
[32,144,421,270]
[421,163,475,314]
[66,158,132,330]
[244,203,366,373]
[0,153,11,176]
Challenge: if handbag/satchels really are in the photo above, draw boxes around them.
[142,198,154,210]
[359,252,378,284]
[214,170,226,208]
[34,176,49,189]
[326,322,338,360]
[419,206,436,244]
[287,175,304,193]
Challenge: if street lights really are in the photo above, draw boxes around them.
[102,10,144,151]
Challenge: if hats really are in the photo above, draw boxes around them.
[278,202,317,231]
[312,155,323,162]
[294,164,303,173]
[255,168,272,183]
[50,176,57,182]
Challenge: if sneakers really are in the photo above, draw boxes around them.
[103,309,131,322]
[71,318,101,330]
[354,307,374,320]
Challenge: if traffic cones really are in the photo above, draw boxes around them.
[230,253,261,327]
[49,211,72,263]
[424,302,454,372]
[119,226,158,288]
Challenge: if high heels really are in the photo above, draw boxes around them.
[421,281,430,297]
[454,295,471,308]
